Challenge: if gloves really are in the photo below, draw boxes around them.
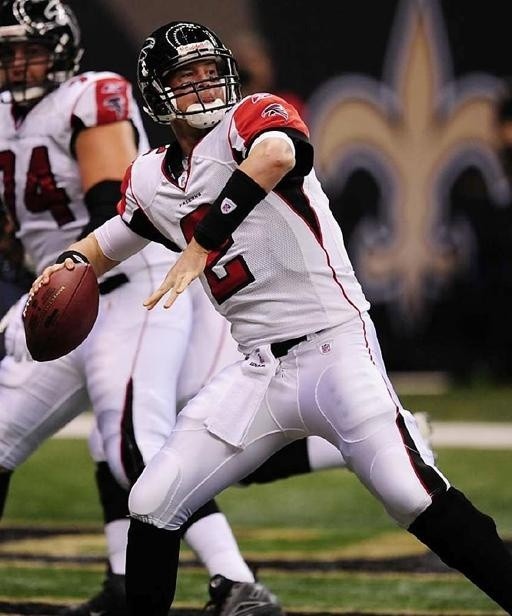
[0,295,35,363]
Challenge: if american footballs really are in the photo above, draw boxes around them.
[22,264,99,362]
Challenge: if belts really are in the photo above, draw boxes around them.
[97,273,130,294]
[245,328,326,363]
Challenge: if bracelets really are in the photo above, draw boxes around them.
[54,250,91,265]
[191,166,270,252]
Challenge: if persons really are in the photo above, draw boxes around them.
[68,272,436,616]
[0,0,289,616]
[27,20,512,614]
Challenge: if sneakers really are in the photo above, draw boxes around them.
[61,561,129,613]
[205,574,282,615]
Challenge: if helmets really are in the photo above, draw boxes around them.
[137,19,235,126]
[0,0,83,81]
